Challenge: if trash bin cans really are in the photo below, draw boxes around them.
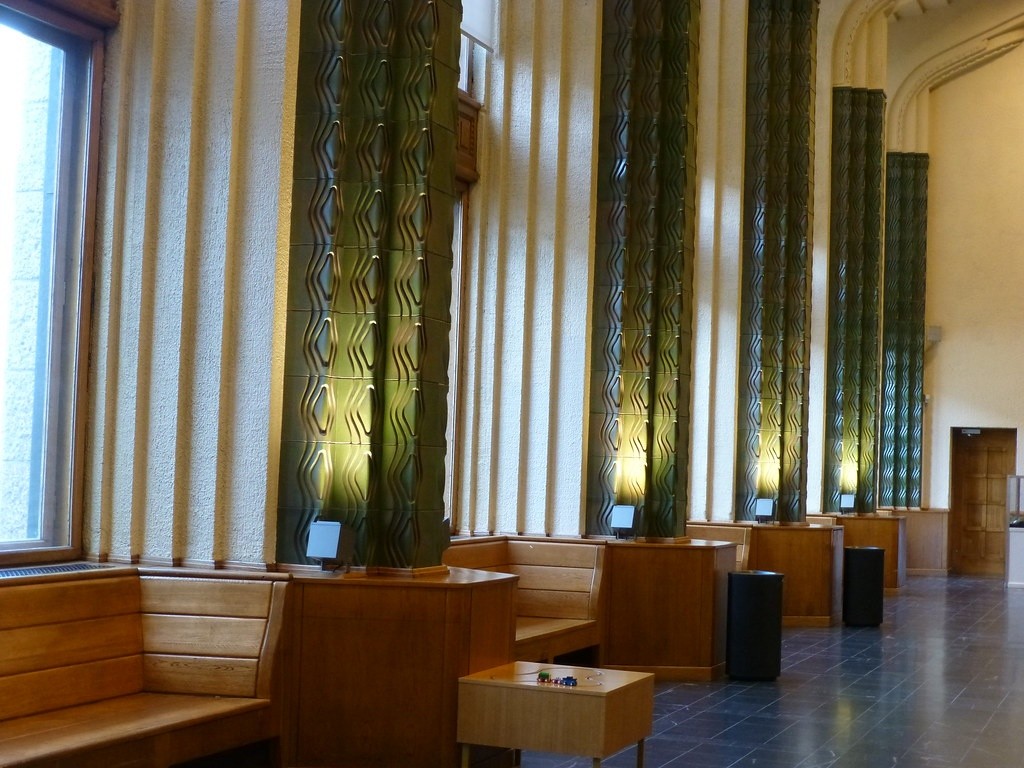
[842,546,885,627]
[726,570,785,681]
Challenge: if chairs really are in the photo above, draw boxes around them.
[441,534,610,672]
[0,561,292,768]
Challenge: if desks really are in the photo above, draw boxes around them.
[457,660,655,768]
